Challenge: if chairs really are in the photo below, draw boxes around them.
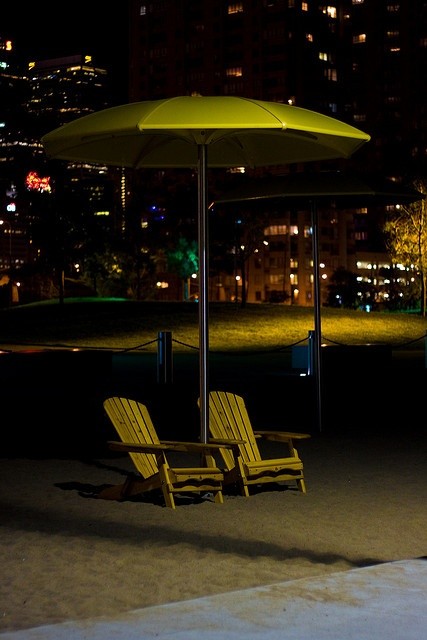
[195,391,311,498]
[102,397,228,509]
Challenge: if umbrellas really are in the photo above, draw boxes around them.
[207,171,426,433]
[40,93,372,467]
